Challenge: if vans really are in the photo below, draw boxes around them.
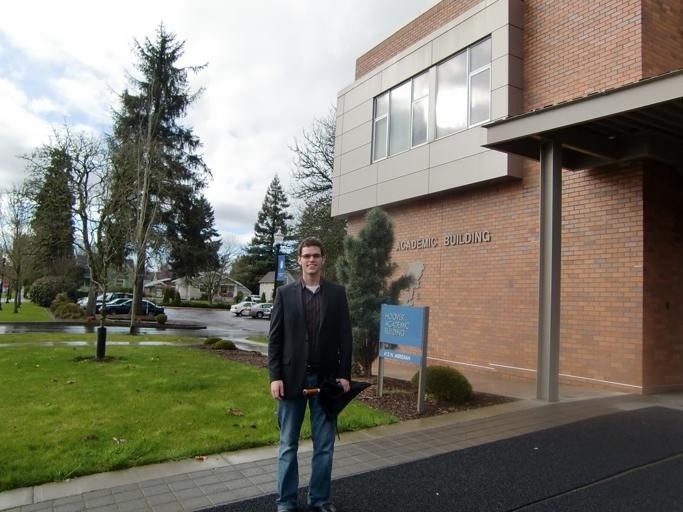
[245,295,261,303]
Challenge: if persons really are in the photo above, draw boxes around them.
[268,237,353,511]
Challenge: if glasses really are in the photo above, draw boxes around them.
[302,254,322,259]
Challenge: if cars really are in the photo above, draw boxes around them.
[242,303,274,319]
[229,301,258,317]
[74,292,164,316]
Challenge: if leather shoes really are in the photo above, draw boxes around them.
[320,503,336,512]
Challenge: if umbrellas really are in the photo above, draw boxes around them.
[301,378,378,441]
